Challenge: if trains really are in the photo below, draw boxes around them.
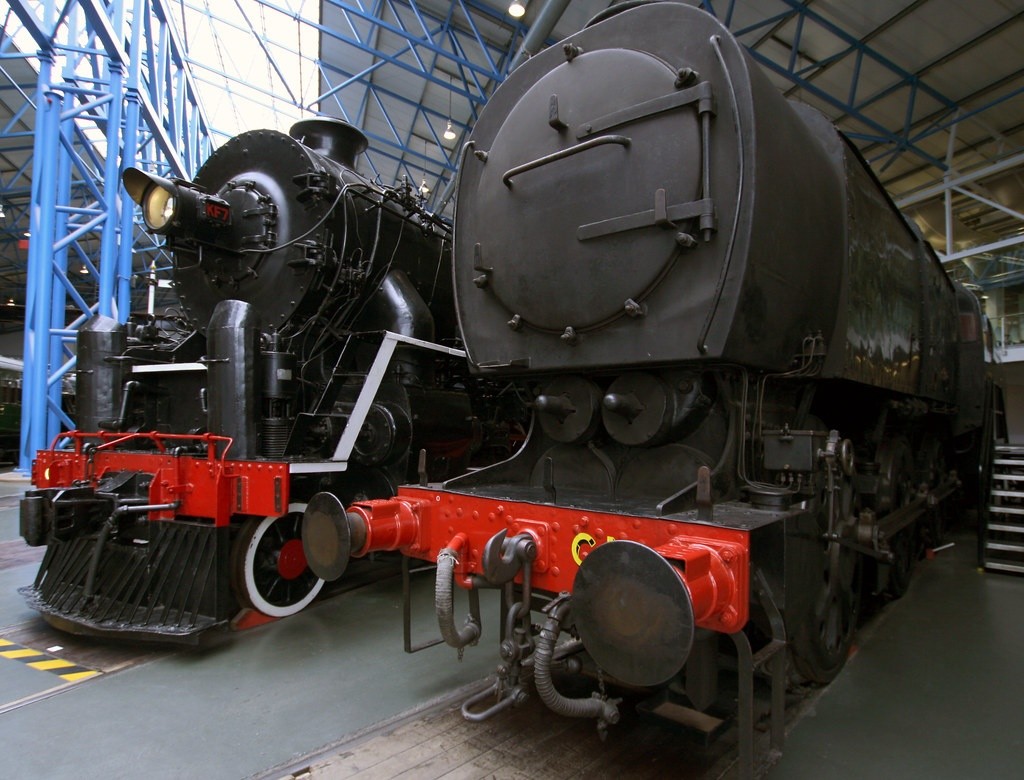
[16,112,532,652]
[300,0,1002,758]
